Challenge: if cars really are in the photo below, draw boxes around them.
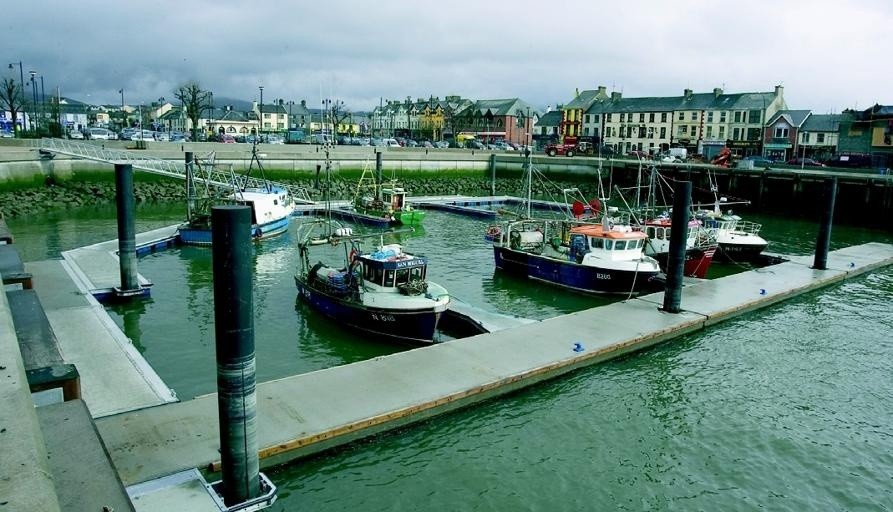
[740,155,827,167]
[63,123,110,143]
[629,142,691,165]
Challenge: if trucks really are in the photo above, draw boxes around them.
[544,135,592,156]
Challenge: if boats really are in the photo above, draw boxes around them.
[289,221,451,343]
[313,167,425,229]
[176,183,292,246]
[493,193,770,299]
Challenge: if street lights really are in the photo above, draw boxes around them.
[118,87,124,125]
[8,62,26,132]
[257,86,265,139]
